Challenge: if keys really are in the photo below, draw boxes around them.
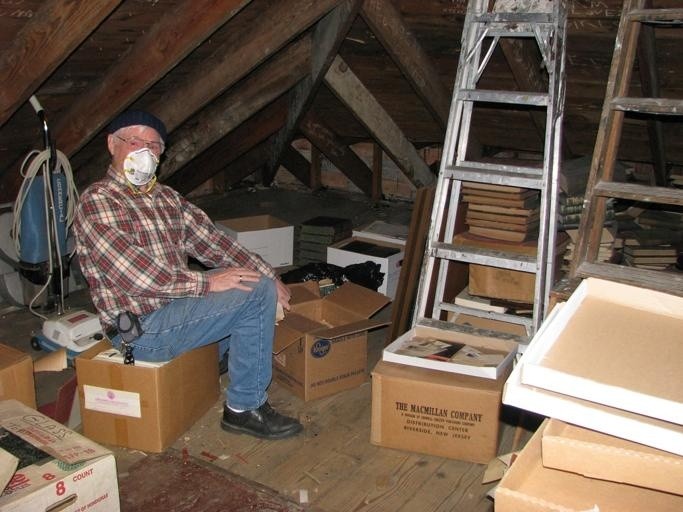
[120,341,128,353]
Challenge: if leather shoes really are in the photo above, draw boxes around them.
[216,349,228,376]
[221,398,303,440]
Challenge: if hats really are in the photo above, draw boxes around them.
[108,109,168,143]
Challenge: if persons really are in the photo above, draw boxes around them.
[69,106,301,439]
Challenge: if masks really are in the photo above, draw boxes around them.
[125,148,158,185]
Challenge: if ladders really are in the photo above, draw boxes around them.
[569,0,683,300]
[411,0,568,355]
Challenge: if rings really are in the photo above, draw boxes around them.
[240,275,242,283]
